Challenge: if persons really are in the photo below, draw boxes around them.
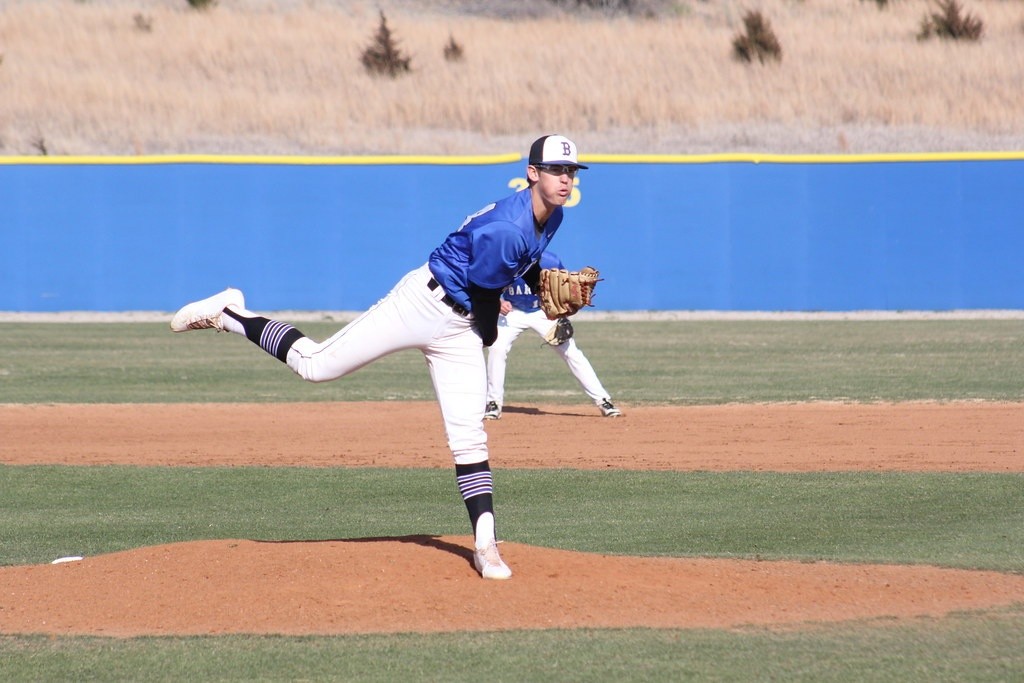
[484,249,620,420]
[170,136,590,581]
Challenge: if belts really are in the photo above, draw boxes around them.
[427,278,469,317]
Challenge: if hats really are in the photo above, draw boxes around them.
[529,134,588,170]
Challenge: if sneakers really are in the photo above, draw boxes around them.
[483,401,502,419]
[170,289,245,334]
[599,398,621,417]
[473,541,511,579]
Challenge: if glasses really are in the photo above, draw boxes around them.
[533,164,579,176]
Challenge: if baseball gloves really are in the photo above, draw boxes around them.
[536,264,598,316]
[548,317,573,346]
